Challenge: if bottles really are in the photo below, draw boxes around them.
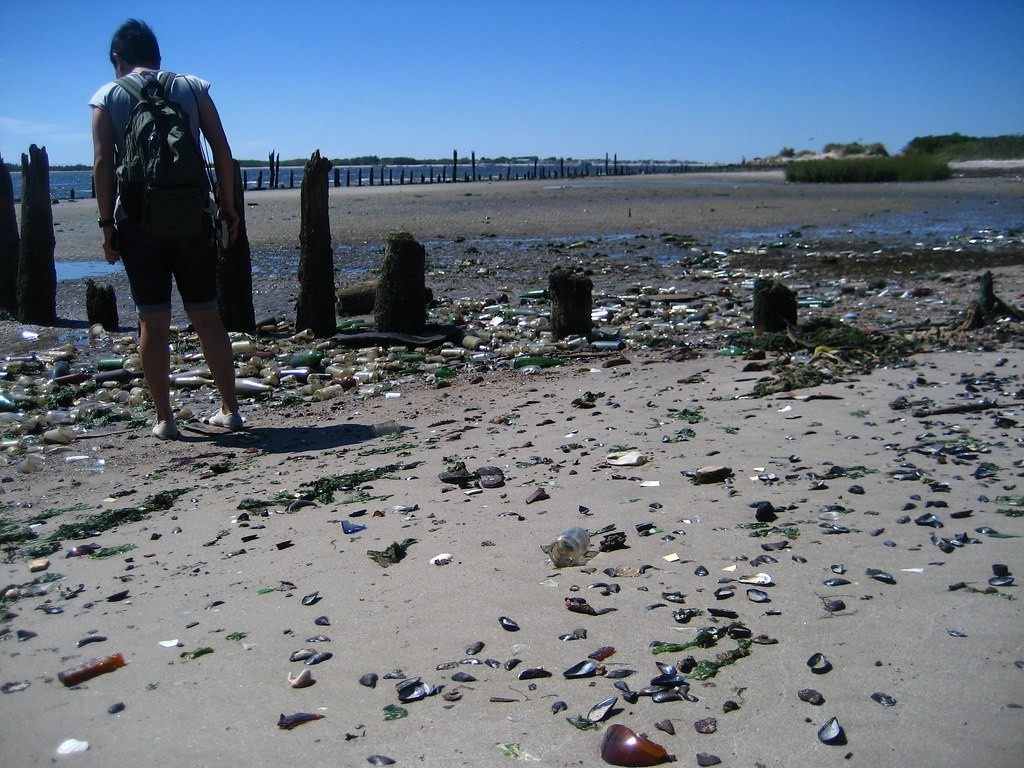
[0,259,829,400]
[549,527,591,567]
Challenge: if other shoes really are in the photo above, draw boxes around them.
[209,408,243,427]
[152,420,177,438]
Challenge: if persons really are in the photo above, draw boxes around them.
[91,17,244,442]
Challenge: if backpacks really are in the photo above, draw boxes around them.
[116,72,211,237]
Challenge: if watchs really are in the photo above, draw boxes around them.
[97,216,115,228]
